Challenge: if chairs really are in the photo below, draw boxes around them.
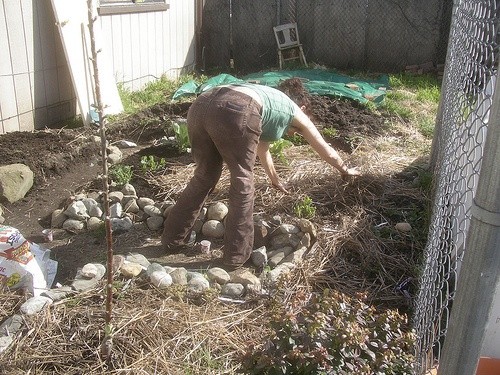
[273,23,308,69]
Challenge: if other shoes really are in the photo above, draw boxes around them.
[187,230,196,244]
[226,264,242,270]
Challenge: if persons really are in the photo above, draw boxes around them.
[160,77,364,271]
